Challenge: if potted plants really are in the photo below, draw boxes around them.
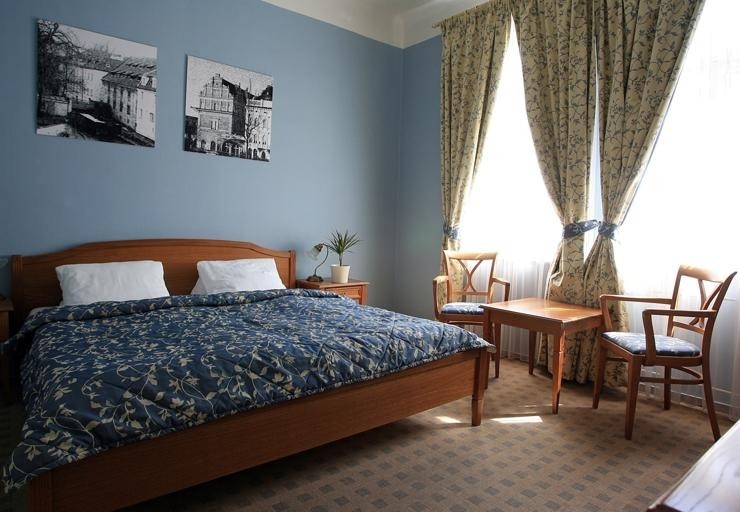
[320,225,364,284]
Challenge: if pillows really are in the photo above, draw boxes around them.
[196,257,287,295]
[54,260,170,306]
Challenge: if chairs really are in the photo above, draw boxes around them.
[433,248,510,378]
[592,264,738,442]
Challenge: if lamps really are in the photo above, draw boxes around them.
[306,243,328,282]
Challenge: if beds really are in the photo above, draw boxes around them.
[1,237,488,511]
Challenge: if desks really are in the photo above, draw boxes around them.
[479,297,614,415]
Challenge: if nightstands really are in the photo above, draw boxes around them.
[296,277,370,305]
